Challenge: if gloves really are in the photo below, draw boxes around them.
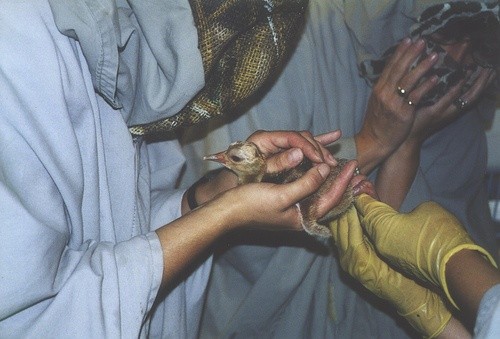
[354,193,498,311]
[329,204,452,339]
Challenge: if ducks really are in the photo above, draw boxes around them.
[201,141,370,240]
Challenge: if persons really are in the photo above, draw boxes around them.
[0,0,500,339]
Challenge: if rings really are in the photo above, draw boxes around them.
[397,86,408,98]
[454,97,471,115]
[406,99,418,107]
